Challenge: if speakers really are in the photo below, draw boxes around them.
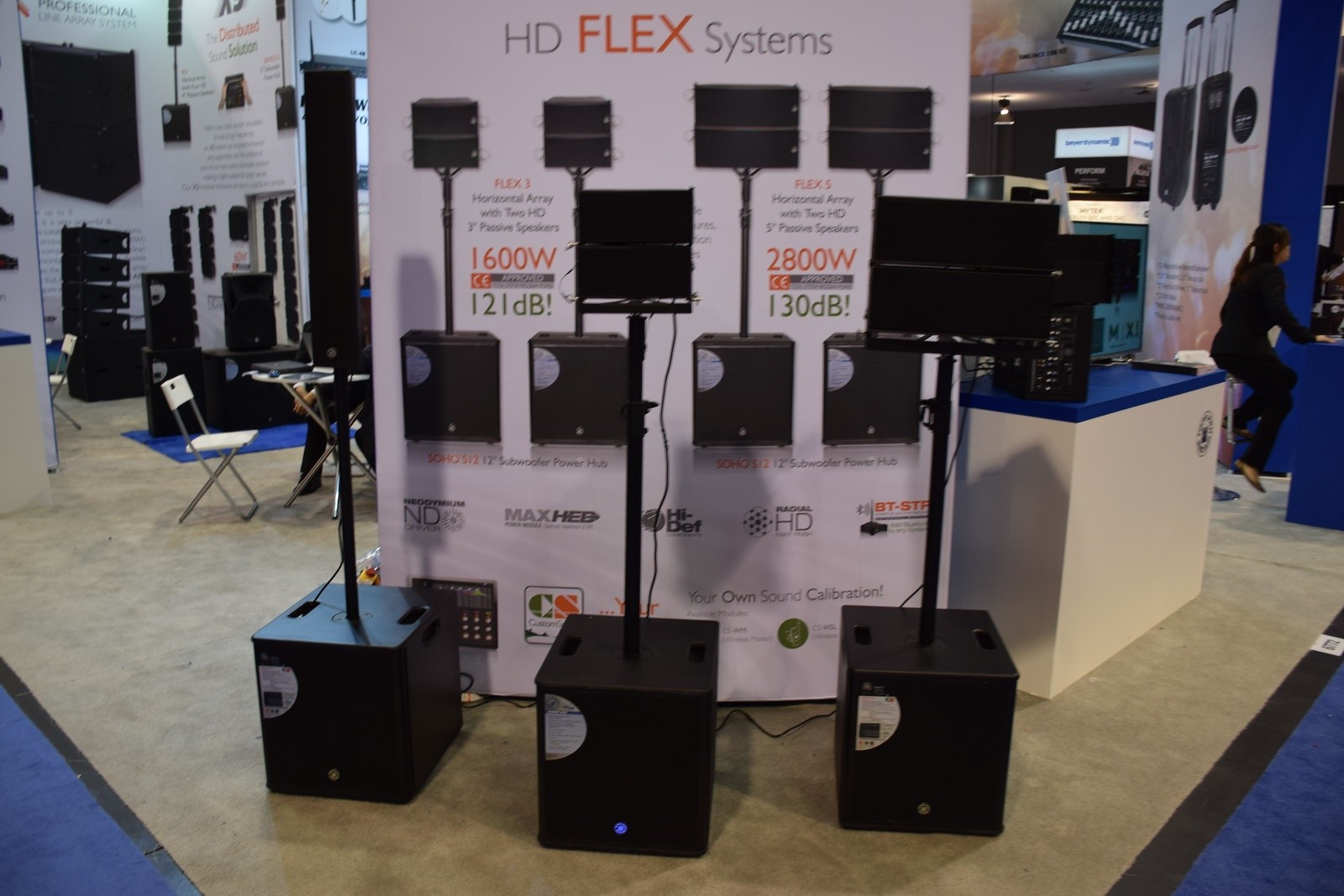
[1158,71,1234,208]
[410,97,479,168]
[168,198,298,341]
[400,330,501,444]
[252,582,463,806]
[60,225,301,437]
[276,85,297,130]
[543,97,613,167]
[161,104,191,140]
[835,605,1018,836]
[276,0,285,20]
[868,194,1116,404]
[533,613,721,857]
[829,86,933,171]
[692,333,795,446]
[823,333,923,446]
[304,70,361,370]
[579,188,692,300]
[528,331,629,446]
[22,40,139,204]
[1115,239,1142,293]
[694,85,801,168]
[168,0,182,46]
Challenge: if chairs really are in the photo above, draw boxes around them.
[48,333,82,430]
[160,373,260,524]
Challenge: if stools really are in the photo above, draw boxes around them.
[1174,349,1249,503]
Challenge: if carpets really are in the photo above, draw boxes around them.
[120,421,356,463]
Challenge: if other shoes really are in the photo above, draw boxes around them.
[1234,460,1266,492]
[1222,420,1253,440]
[294,470,321,493]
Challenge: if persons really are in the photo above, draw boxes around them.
[1208,223,1337,494]
[292,318,358,496]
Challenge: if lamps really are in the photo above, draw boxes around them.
[993,96,1015,126]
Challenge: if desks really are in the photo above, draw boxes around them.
[957,365,1226,700]
[253,366,376,520]
[1287,340,1344,531]
